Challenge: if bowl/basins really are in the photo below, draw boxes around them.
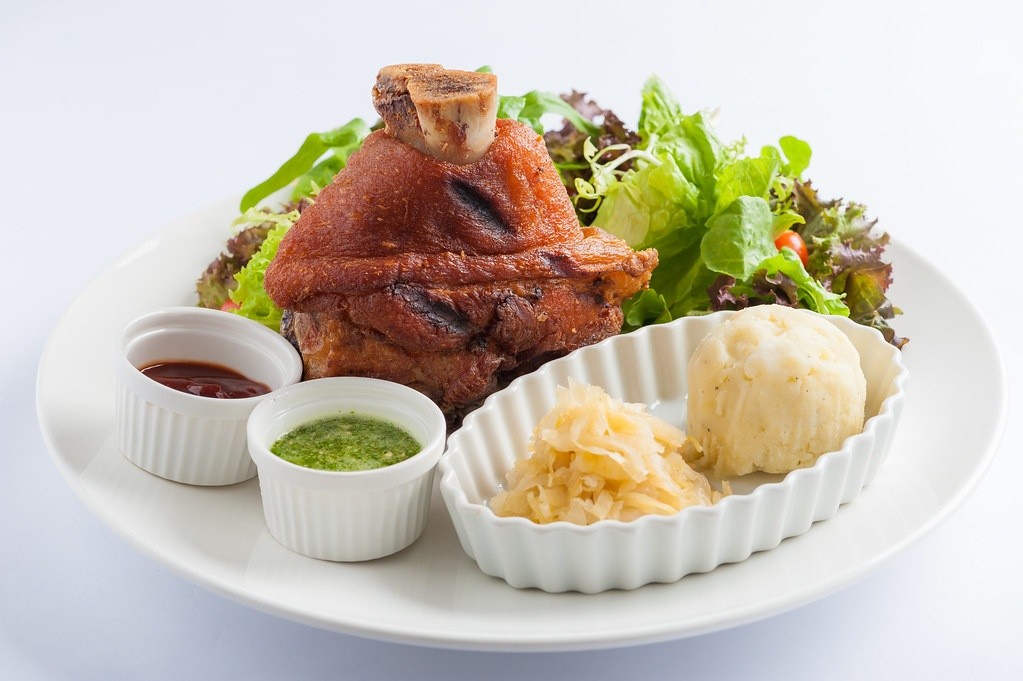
[245,377,447,564]
[438,303,905,599]
[117,308,303,488]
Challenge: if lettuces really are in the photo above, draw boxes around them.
[193,63,912,433]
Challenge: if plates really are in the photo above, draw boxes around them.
[37,159,1005,659]
